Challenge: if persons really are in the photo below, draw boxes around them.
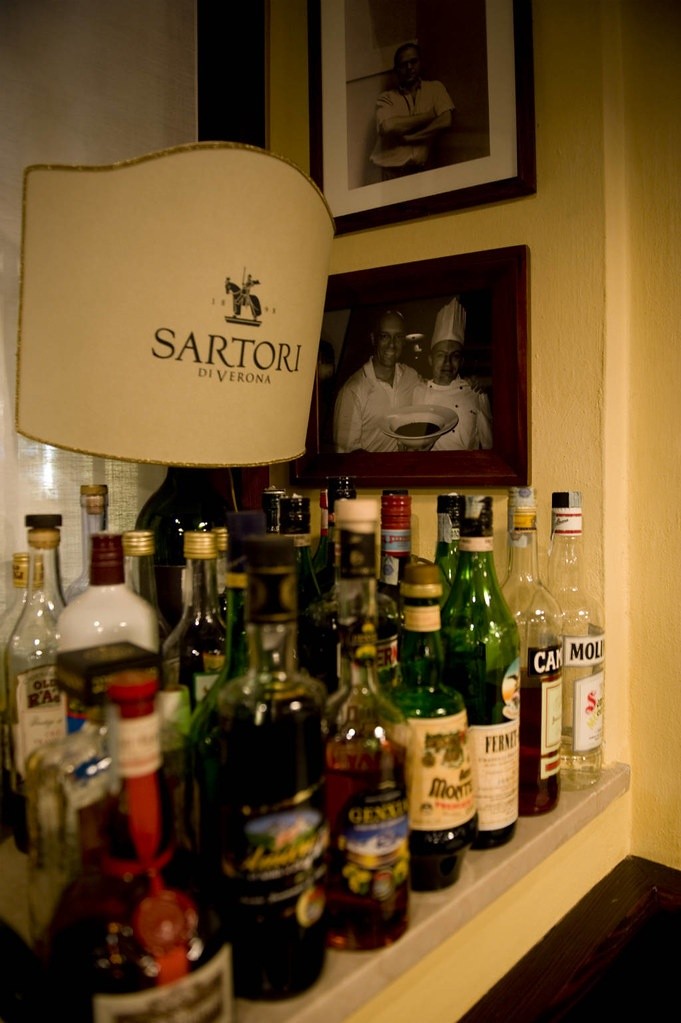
[333,311,488,453]
[370,43,456,182]
[413,297,493,451]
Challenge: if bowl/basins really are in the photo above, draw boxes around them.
[379,405,459,450]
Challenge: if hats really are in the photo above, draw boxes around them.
[430,299,467,351]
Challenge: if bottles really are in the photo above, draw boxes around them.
[0,466,607,1023]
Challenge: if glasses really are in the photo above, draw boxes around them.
[375,333,407,343]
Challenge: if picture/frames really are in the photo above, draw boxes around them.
[306,0,537,239]
[290,243,532,490]
